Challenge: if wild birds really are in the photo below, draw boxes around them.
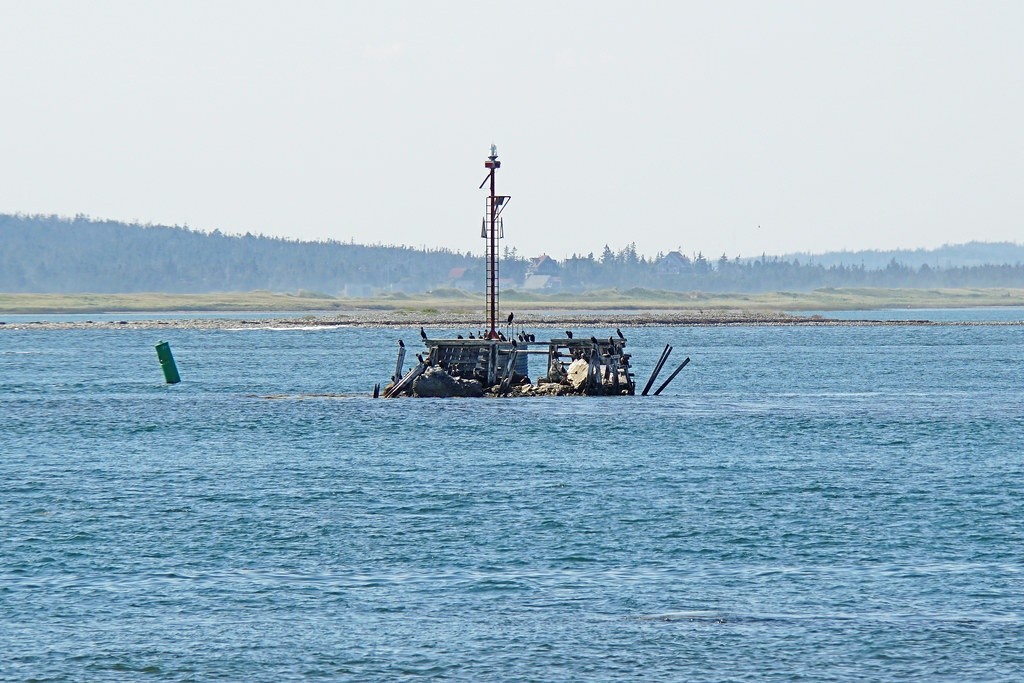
[457,329,535,348]
[398,339,405,349]
[573,336,630,365]
[552,348,559,362]
[566,330,573,339]
[617,328,624,340]
[507,312,514,325]
[415,353,425,364]
[420,326,428,340]
